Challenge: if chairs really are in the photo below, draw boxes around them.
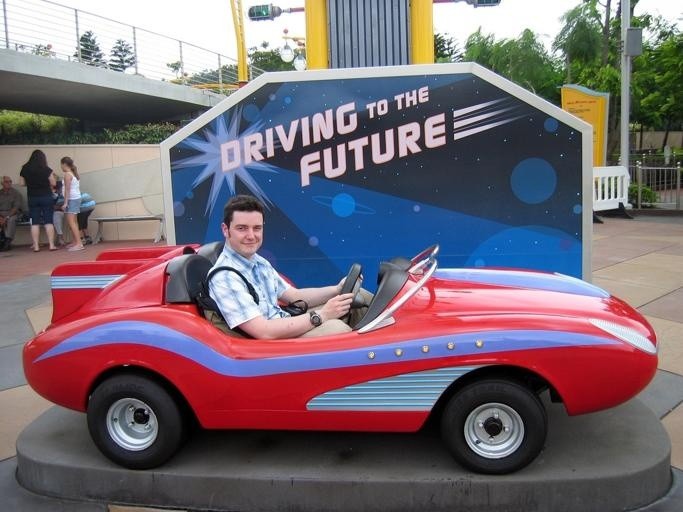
[164,241,226,320]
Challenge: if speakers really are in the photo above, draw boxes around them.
[624,27,642,57]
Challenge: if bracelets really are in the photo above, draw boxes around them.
[5,215,9,219]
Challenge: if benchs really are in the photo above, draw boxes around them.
[88,215,164,245]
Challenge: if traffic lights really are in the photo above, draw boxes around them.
[248,3,305,20]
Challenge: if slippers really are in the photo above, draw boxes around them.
[34,248,60,252]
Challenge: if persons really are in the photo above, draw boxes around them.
[28,173,67,249]
[0,175,24,252]
[54,180,96,245]
[18,149,62,252]
[60,156,86,252]
[204,195,375,342]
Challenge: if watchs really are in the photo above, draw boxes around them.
[310,311,322,327]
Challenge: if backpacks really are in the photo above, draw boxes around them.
[195,267,262,341]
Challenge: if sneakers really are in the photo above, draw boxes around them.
[65,235,94,253]
